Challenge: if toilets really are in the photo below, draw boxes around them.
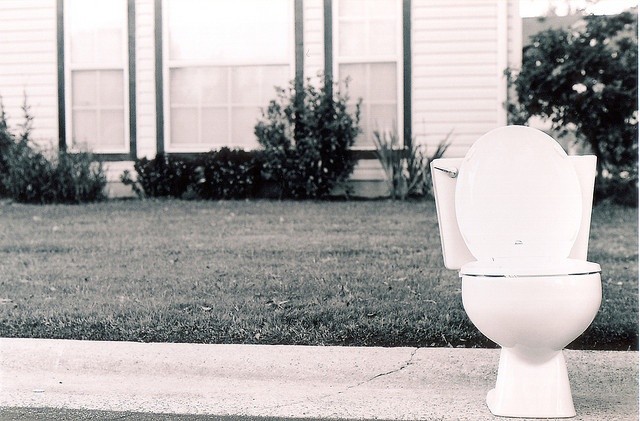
[429,125,603,419]
